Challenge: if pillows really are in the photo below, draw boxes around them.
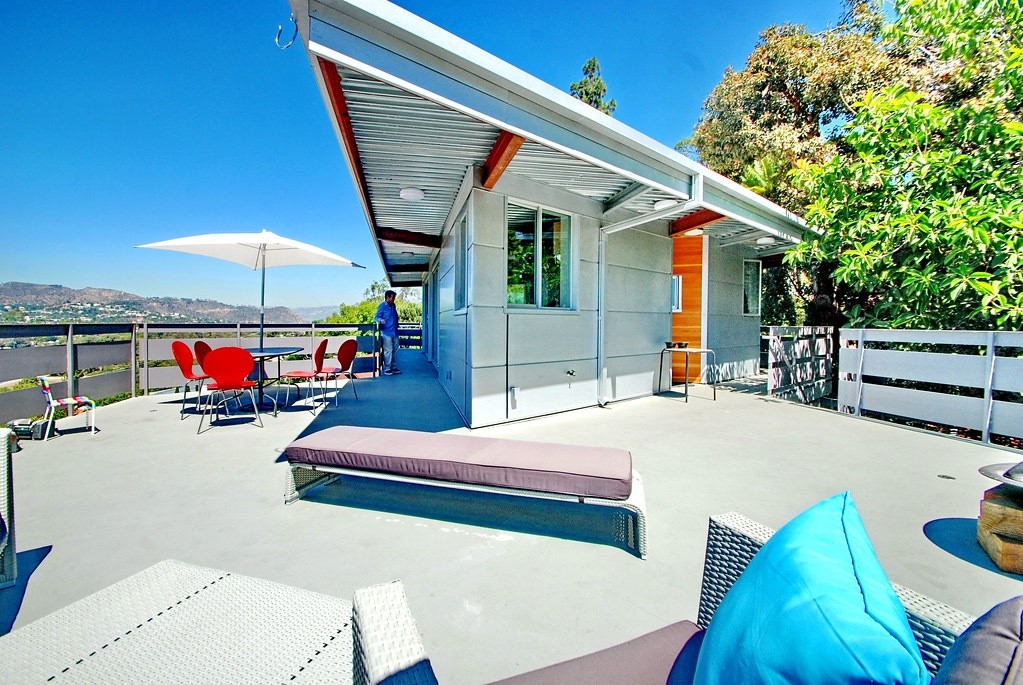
[690,491,930,685]
[932,594,1023,685]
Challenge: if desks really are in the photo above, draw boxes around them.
[239,346,305,412]
[0,559,353,685]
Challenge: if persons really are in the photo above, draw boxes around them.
[374,290,401,376]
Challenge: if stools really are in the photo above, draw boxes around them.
[658,348,713,404]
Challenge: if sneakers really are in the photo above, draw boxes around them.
[384,369,393,375]
[391,367,401,373]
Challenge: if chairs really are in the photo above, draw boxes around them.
[40,377,96,441]
[171,338,359,435]
[351,507,979,685]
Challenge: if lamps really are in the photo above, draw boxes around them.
[757,237,776,245]
[653,199,678,211]
[684,229,703,237]
[400,252,414,257]
[399,188,426,203]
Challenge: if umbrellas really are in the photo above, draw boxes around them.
[130,228,366,405]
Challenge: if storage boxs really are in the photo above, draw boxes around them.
[984,483,1023,500]
[980,498,1023,542]
[976,522,1023,575]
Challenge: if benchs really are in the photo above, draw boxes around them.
[275,424,650,562]
